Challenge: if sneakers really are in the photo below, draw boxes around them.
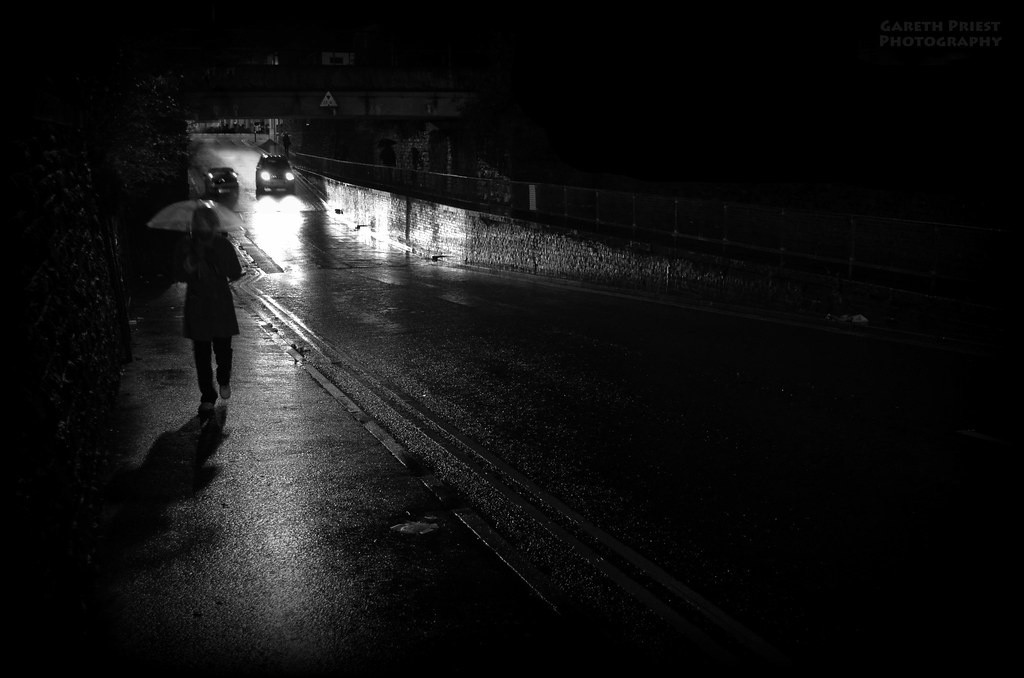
[200,401,214,411]
[220,383,230,399]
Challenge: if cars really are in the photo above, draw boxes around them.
[205,167,239,200]
[256,154,295,193]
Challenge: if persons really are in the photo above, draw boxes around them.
[283,134,290,155]
[178,207,242,411]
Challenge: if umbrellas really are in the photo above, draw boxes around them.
[149,199,243,230]
